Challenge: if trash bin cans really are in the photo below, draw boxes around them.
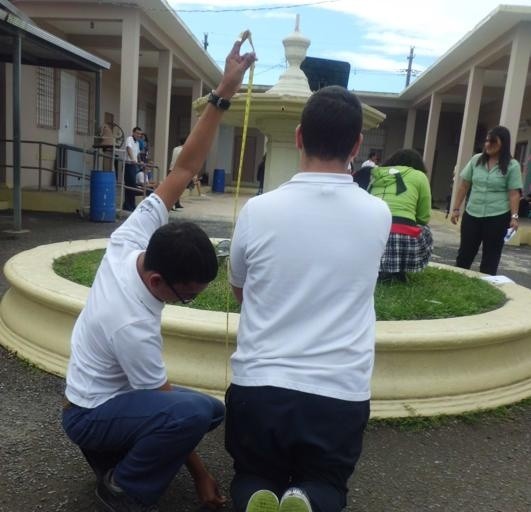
[90,171,116,223]
[212,168,224,193]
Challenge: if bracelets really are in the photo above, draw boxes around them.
[452,208,459,211]
[205,89,230,110]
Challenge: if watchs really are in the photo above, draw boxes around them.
[510,213,519,219]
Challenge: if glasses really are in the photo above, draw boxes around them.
[163,276,196,303]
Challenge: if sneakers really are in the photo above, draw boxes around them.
[95,469,143,511]
[247,487,311,512]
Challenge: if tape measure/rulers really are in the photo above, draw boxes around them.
[222,30,254,508]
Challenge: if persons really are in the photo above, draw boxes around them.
[347,148,433,283]
[257,152,266,196]
[65,27,258,512]
[222,83,392,512]
[123,125,208,212]
[519,193,531,219]
[449,124,523,275]
